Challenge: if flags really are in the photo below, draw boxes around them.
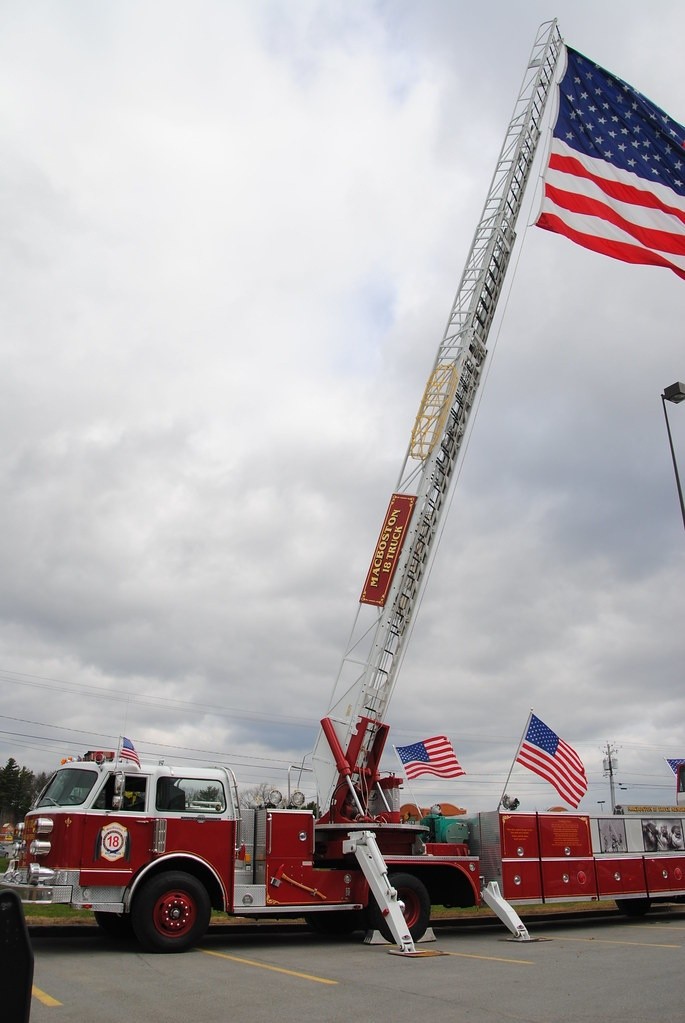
[666,759,685,778]
[395,736,466,780]
[120,737,141,768]
[516,714,589,809]
[535,44,685,280]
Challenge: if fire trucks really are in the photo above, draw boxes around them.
[0,15,685,959]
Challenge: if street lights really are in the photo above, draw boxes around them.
[659,381,685,521]
